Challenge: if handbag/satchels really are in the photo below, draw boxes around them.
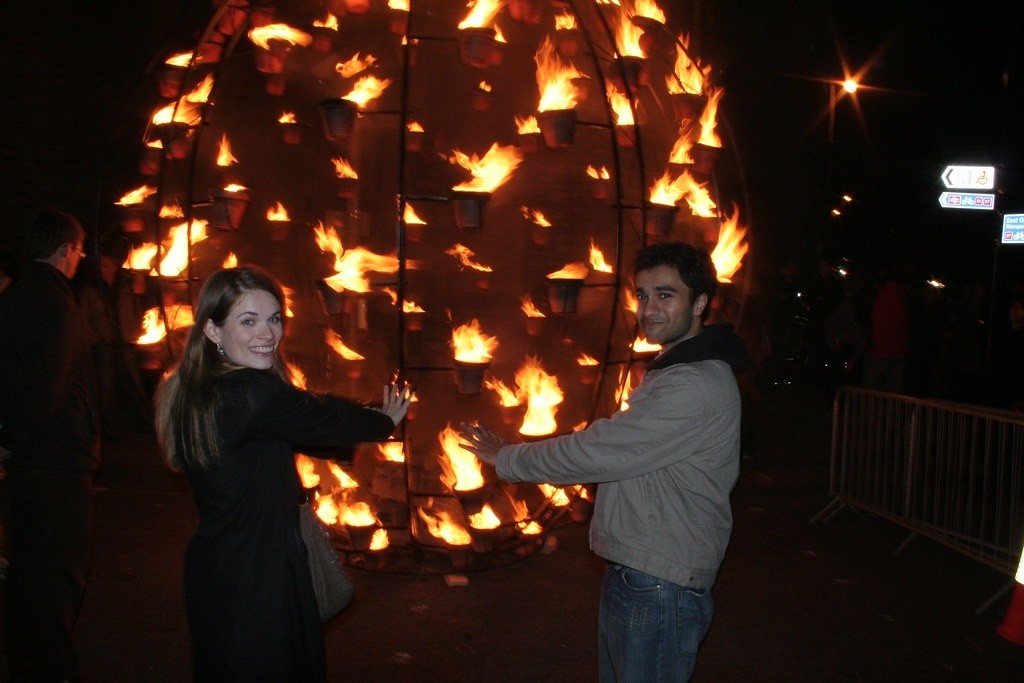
[299,504,353,623]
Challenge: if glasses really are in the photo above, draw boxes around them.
[63,244,88,260]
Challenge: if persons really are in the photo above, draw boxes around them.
[776,255,1024,413]
[458,243,747,683]
[154,267,416,683]
[0,206,154,631]
